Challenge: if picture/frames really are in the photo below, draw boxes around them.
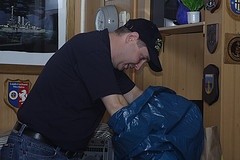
[0,0,67,66]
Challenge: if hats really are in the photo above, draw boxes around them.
[125,18,163,72]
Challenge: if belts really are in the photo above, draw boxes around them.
[13,121,85,159]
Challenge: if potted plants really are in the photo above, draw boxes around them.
[181,0,205,25]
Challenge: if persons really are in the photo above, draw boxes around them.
[0,18,164,160]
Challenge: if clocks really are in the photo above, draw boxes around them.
[94,5,119,33]
